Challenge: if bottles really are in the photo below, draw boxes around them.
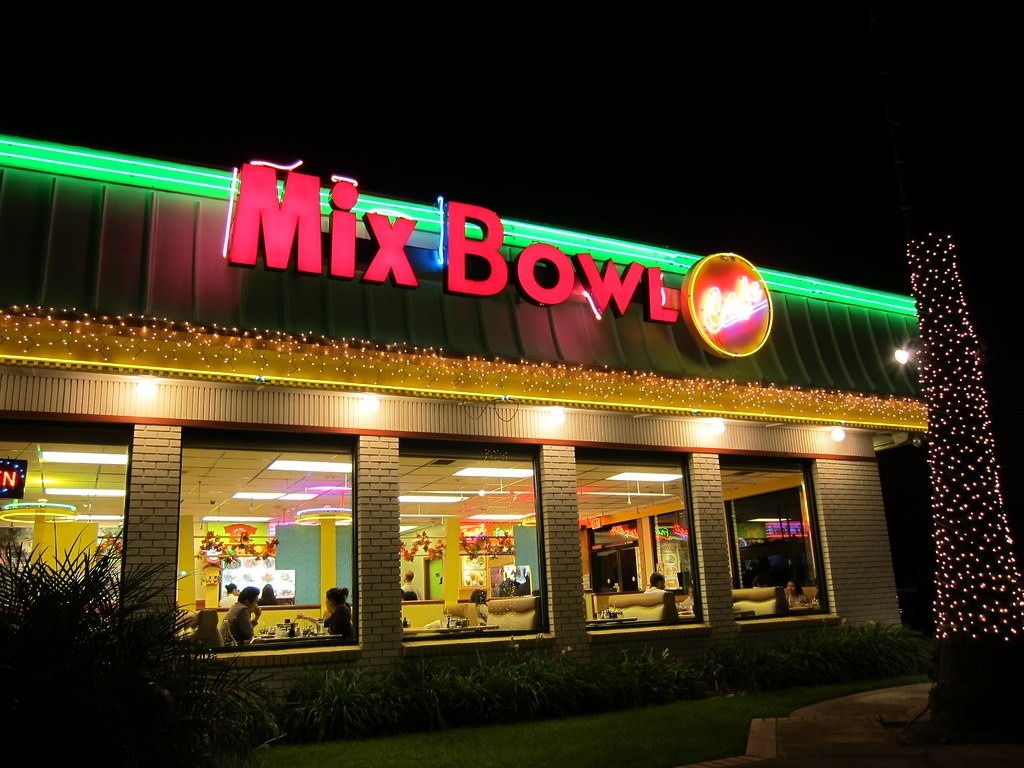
[295,627,300,636]
[403,618,408,628]
[315,619,324,637]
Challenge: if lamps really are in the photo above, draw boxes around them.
[895,341,916,364]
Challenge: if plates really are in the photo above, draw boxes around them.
[254,635,275,638]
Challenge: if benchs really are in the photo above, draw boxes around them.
[731,586,790,619]
[486,596,541,631]
[446,603,478,627]
[194,610,226,648]
[591,591,678,621]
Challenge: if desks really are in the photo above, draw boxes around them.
[790,607,815,611]
[585,616,637,624]
[404,625,500,634]
[734,610,755,618]
[254,634,341,642]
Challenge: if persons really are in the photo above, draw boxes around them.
[258,584,279,606]
[220,586,262,645]
[402,569,420,600]
[324,587,352,635]
[218,581,241,608]
[191,609,225,646]
[678,586,694,611]
[470,589,488,626]
[644,572,668,593]
[783,580,811,606]
